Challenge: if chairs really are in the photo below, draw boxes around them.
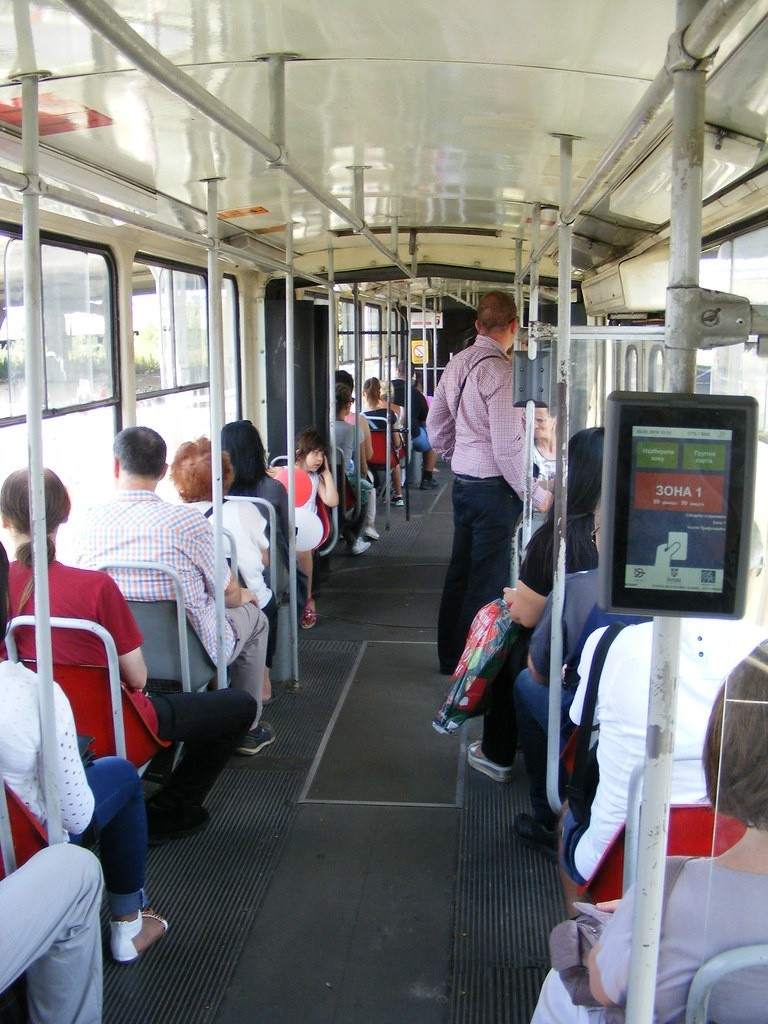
[0,413,768,1024]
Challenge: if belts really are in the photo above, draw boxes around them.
[455,477,506,487]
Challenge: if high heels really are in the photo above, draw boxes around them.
[468,739,512,784]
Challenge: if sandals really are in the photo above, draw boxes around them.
[300,595,316,628]
[108,910,168,963]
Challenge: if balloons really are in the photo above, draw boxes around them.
[274,467,323,551]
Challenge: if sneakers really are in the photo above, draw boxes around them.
[364,526,380,540]
[351,538,371,556]
[421,479,439,490]
[235,721,275,756]
[514,813,561,862]
[390,496,404,506]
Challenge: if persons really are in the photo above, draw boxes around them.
[391,360,439,490]
[265,425,339,629]
[513,499,602,862]
[425,291,554,676]
[335,382,379,556]
[169,432,282,704]
[0,428,276,1023]
[335,369,374,485]
[467,426,605,778]
[522,408,555,538]
[377,379,406,444]
[362,377,404,506]
[529,516,768,1024]
[221,419,308,630]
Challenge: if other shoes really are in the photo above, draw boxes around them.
[144,799,209,845]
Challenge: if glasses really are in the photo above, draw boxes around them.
[591,526,603,547]
[345,398,355,406]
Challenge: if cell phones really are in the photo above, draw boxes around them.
[316,465,326,476]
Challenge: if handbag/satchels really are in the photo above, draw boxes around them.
[433,598,521,735]
[549,898,602,1006]
